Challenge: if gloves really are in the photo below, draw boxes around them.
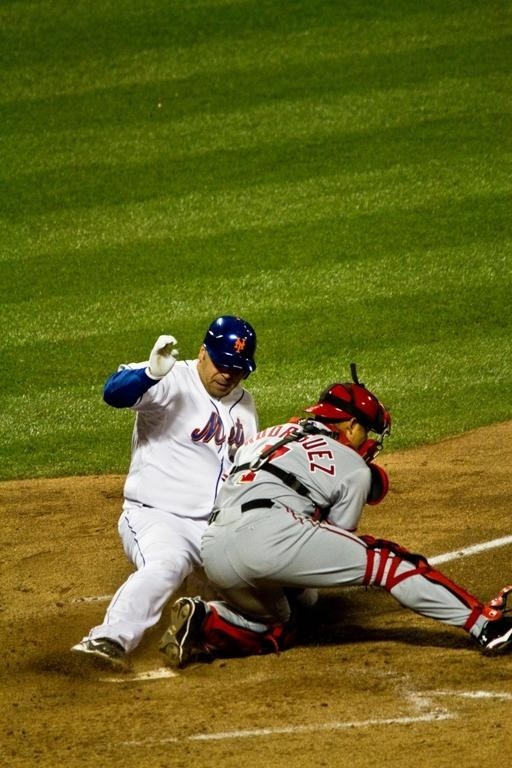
[149,335,179,377]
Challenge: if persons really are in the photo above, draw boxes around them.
[157,379,511,670]
[69,315,260,676]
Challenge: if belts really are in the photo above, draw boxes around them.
[208,497,272,522]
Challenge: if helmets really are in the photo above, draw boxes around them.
[302,382,392,435]
[203,316,257,373]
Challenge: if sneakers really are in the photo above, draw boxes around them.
[71,638,132,673]
[158,596,206,671]
[478,586,511,656]
